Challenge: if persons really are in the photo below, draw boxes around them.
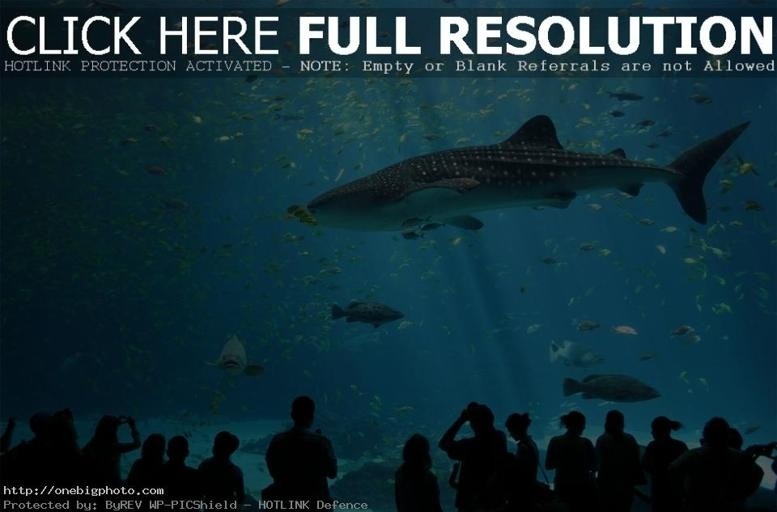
[266,395,336,510]
[0,411,243,501]
[396,403,765,510]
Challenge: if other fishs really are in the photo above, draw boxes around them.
[0,1,777,500]
